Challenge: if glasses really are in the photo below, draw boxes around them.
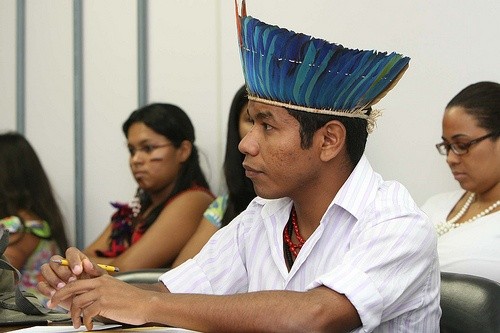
[436,131,497,155]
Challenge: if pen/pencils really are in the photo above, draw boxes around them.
[52,260,119,273]
[0,321,53,327]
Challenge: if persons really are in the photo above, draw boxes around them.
[0,131,68,315]
[79,103,217,275]
[37,98,441,333]
[172,84,258,269]
[419,81,500,283]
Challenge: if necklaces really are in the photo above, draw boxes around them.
[283,206,306,271]
[437,192,500,234]
